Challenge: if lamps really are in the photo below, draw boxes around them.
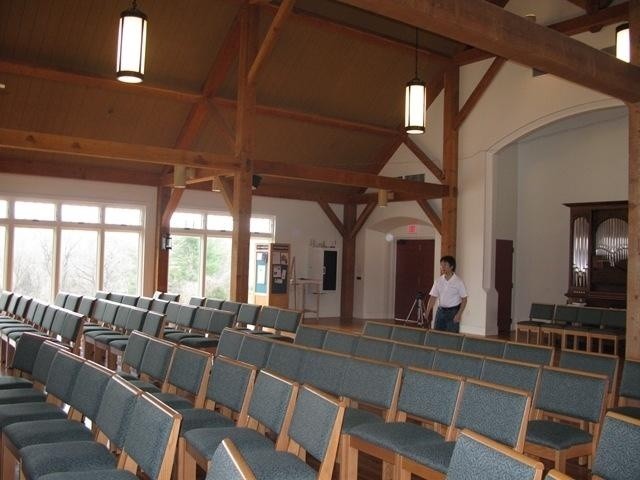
[174,166,186,189]
[405,29,427,135]
[252,175,262,190]
[114,1,148,83]
[212,180,221,192]
[161,232,172,251]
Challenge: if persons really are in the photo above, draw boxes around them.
[424,255,468,334]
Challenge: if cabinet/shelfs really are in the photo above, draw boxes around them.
[309,247,339,293]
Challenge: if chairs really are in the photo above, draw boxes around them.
[0,291,640,480]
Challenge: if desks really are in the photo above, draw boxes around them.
[290,278,323,321]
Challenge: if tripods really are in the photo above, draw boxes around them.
[403,292,431,329]
